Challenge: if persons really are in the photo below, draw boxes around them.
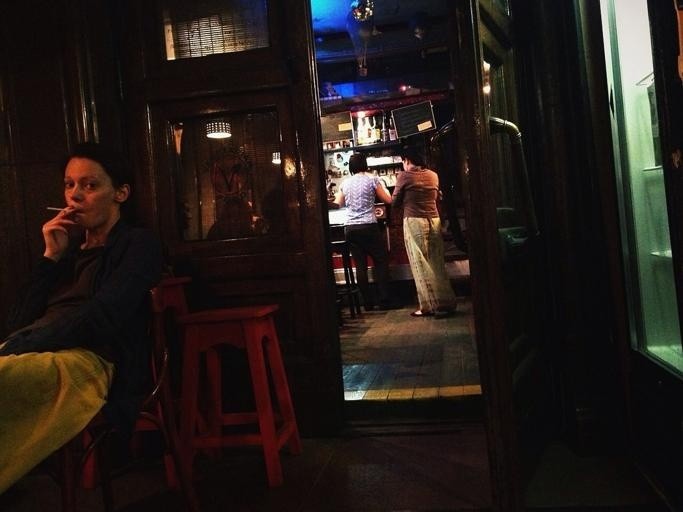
[394,150,456,319]
[0,143,152,428]
[336,154,393,309]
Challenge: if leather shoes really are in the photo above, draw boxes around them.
[410,310,432,316]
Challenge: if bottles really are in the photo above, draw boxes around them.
[357,115,396,145]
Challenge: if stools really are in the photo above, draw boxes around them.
[177,303,302,491]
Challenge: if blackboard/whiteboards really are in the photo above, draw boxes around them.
[391,100,437,140]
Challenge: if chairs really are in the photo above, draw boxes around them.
[73,271,187,492]
